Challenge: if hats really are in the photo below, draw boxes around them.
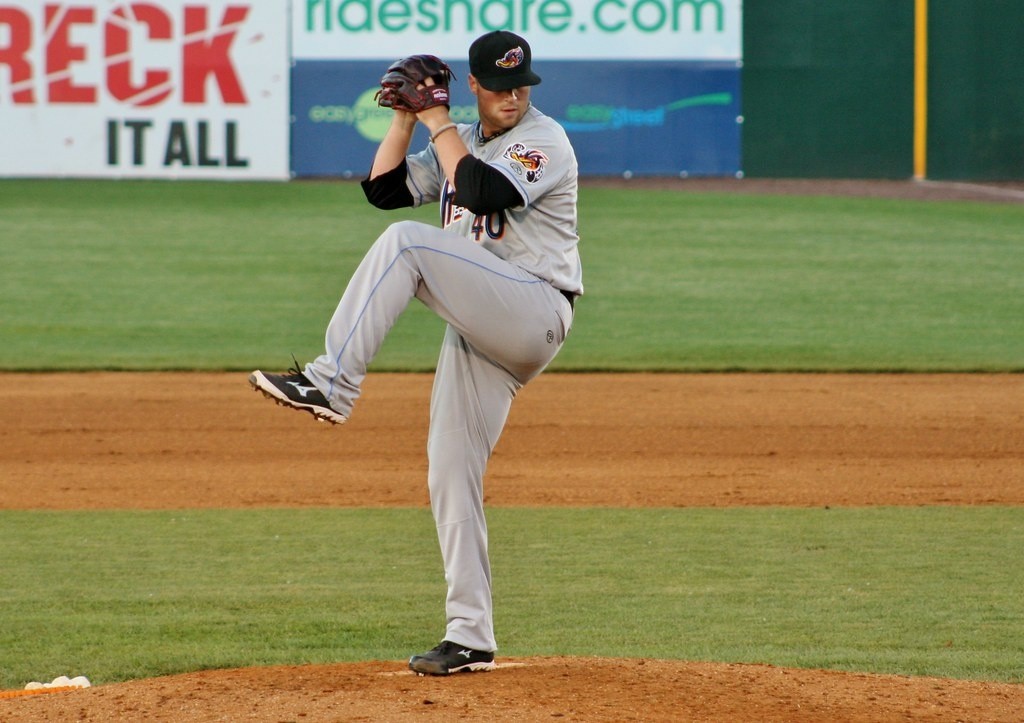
[468,30,541,92]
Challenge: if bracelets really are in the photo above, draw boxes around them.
[429,122,458,144]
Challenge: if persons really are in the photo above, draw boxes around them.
[247,30,585,677]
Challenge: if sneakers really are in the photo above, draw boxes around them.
[409,640,496,676]
[248,352,347,424]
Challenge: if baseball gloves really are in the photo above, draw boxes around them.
[378,54,452,111]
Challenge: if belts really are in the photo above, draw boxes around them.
[561,289,573,314]
